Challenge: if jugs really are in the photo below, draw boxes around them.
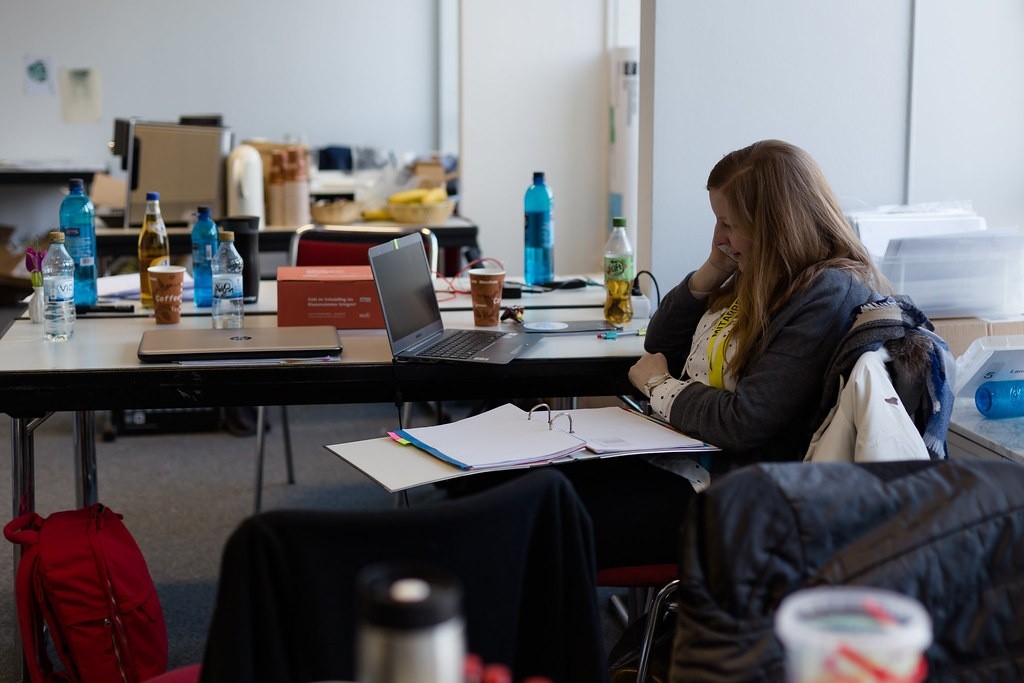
[226,141,266,232]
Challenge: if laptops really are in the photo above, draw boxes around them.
[368,232,543,366]
[137,326,344,363]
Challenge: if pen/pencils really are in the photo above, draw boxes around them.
[630,410,676,430]
[75,304,135,314]
[598,331,639,338]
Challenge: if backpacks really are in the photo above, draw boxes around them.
[3,501,169,682]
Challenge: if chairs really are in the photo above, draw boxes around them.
[141,224,1024,683]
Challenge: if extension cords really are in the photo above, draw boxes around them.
[631,294,651,319]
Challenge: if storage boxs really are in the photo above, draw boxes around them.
[406,162,460,191]
[930,320,1024,360]
[277,266,386,330]
[871,252,1024,319]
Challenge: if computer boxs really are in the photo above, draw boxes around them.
[124,118,235,228]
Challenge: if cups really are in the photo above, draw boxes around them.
[148,264,187,324]
[222,216,260,304]
[468,268,506,326]
[772,584,934,683]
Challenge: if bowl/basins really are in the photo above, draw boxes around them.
[388,198,458,222]
[266,146,311,228]
[313,202,367,223]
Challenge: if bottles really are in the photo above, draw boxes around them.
[604,217,635,324]
[41,231,77,343]
[191,206,220,307]
[210,231,244,328]
[975,379,1024,419]
[524,171,555,286]
[59,178,99,311]
[137,192,170,308]
[351,578,470,683]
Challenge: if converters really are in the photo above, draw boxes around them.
[501,283,523,299]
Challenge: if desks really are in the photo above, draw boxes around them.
[0,159,652,682]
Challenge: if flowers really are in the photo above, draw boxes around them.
[24,232,46,287]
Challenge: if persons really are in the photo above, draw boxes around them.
[444,141,895,575]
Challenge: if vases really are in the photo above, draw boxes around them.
[28,285,44,324]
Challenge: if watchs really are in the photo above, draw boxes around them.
[644,371,672,397]
